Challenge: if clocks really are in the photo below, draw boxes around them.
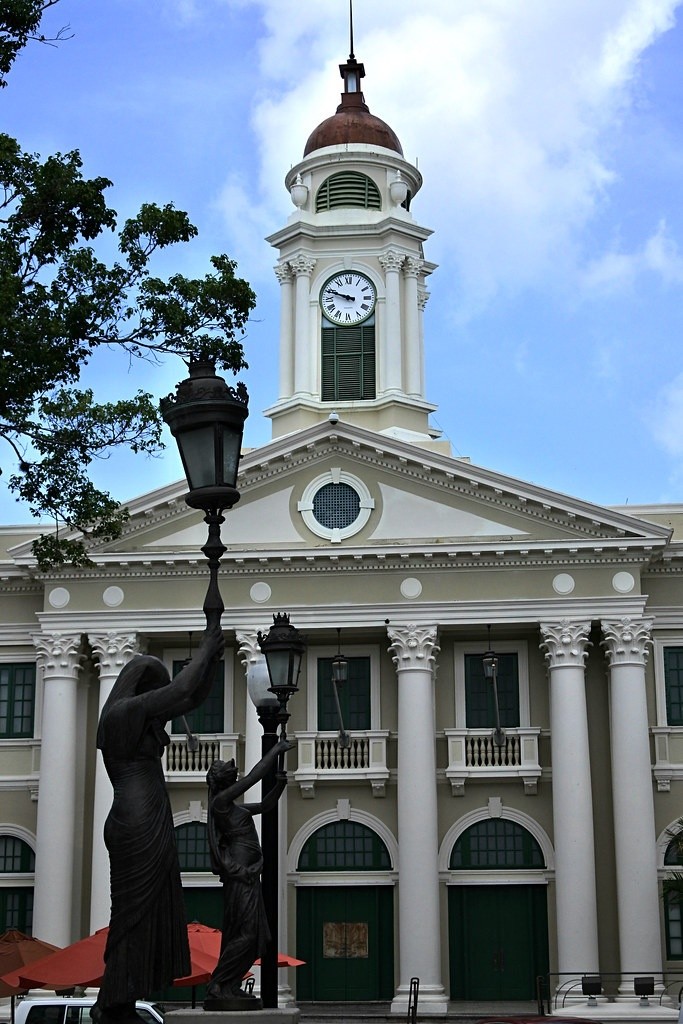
[319,270,378,326]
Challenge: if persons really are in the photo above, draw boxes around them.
[90,625,224,1024]
[205,741,293,1011]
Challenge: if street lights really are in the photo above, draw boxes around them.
[245,643,293,1009]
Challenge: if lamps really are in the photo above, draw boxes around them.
[582,976,603,1006]
[330,628,349,685]
[634,977,657,1006]
[481,624,501,679]
[181,631,194,669]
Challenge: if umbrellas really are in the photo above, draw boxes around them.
[0,919,304,1024]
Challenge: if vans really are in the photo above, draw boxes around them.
[11,987,165,1024]
